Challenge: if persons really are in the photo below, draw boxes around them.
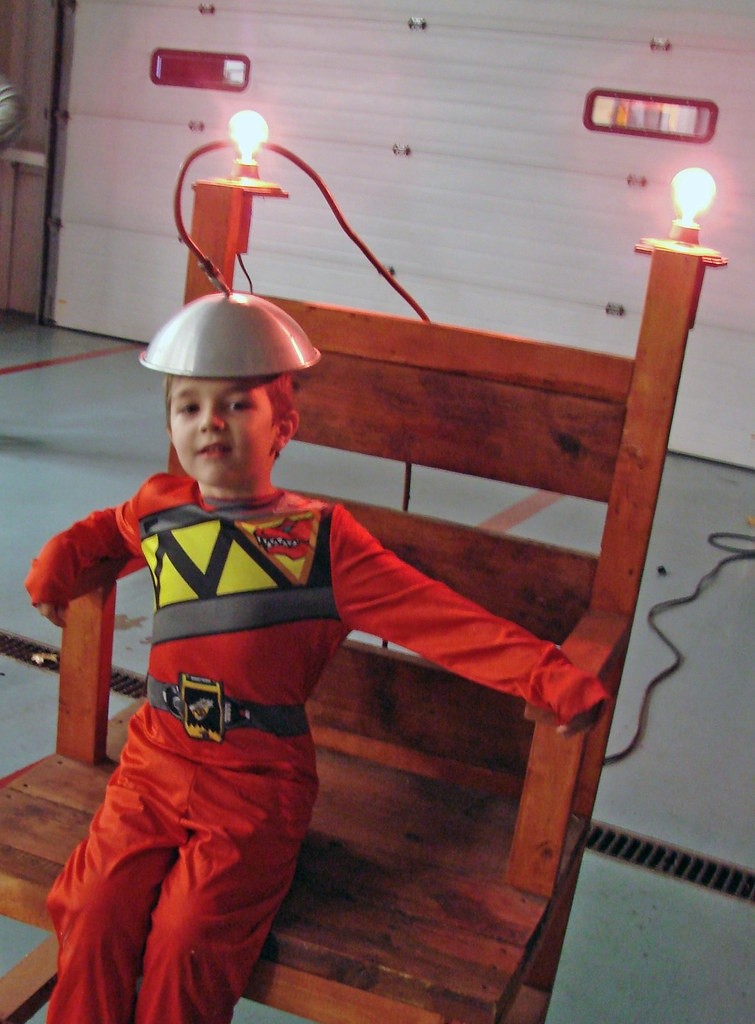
[24,374,610,1024]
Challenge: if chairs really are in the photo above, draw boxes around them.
[0,178,728,1024]
